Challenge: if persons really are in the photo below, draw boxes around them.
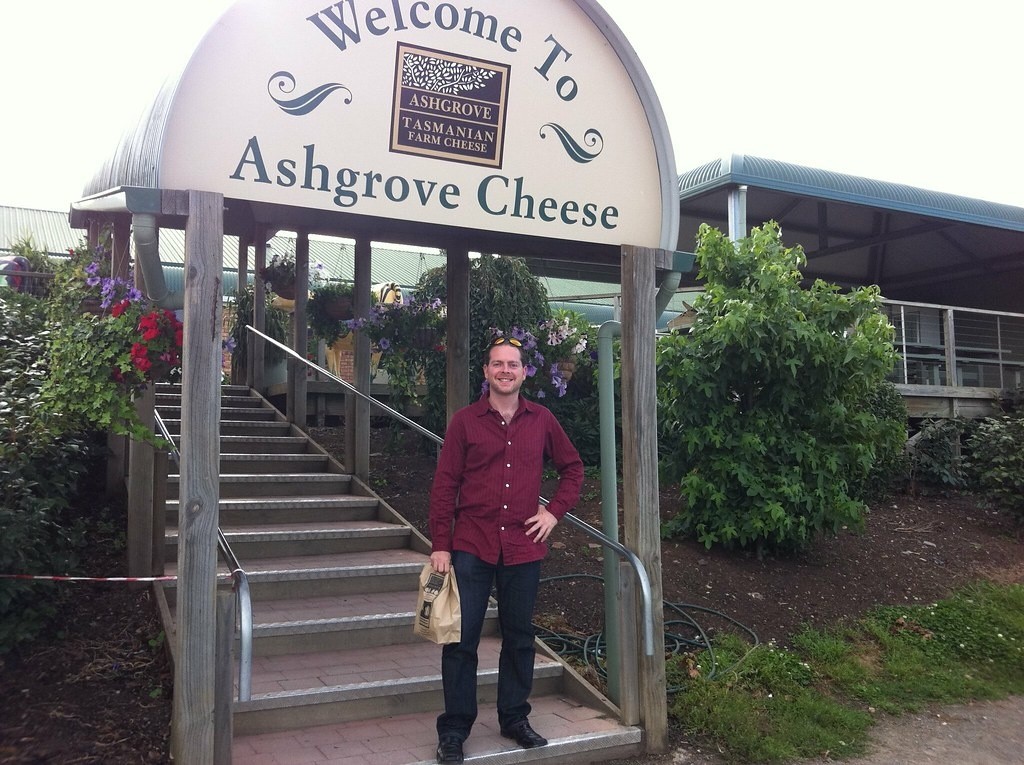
[428,337,583,765]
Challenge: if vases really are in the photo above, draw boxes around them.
[548,354,578,388]
[270,279,296,300]
[409,326,438,351]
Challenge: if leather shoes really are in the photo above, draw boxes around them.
[501,722,547,749]
[436,736,464,765]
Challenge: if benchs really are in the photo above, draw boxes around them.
[894,353,1024,389]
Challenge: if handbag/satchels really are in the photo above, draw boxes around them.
[414,565,461,644]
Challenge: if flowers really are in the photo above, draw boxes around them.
[481,313,588,397]
[257,247,323,289]
[345,296,447,410]
[56,237,238,398]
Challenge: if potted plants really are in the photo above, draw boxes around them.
[303,279,379,348]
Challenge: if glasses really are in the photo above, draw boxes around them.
[492,337,523,348]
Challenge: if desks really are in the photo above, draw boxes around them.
[895,340,1011,387]
